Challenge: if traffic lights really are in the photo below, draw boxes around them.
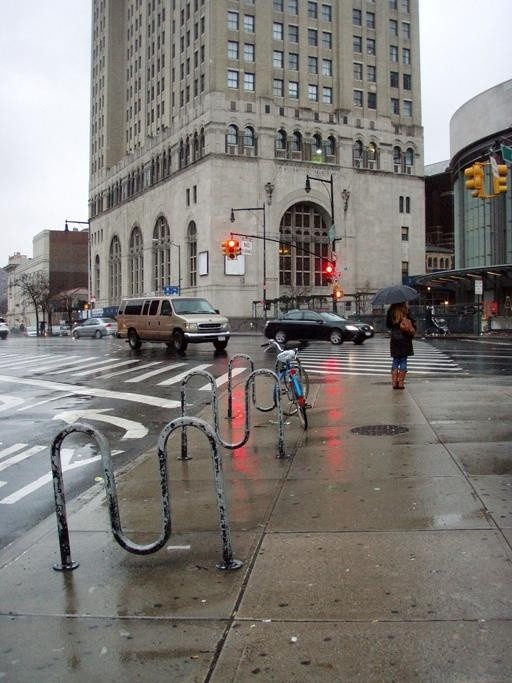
[221,240,241,259]
[333,286,343,299]
[497,163,508,194]
[326,266,333,282]
[464,166,483,197]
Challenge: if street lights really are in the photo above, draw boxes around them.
[230,203,266,301]
[64,220,92,300]
[304,173,337,313]
[152,239,181,296]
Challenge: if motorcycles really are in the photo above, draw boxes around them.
[427,316,449,334]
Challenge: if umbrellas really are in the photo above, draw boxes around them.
[368,281,420,305]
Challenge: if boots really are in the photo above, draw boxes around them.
[392,368,406,389]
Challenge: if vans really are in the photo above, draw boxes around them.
[116,296,232,351]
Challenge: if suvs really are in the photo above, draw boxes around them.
[0,317,9,339]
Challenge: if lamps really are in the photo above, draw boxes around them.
[341,188,351,212]
[264,181,276,205]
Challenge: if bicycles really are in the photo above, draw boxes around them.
[261,339,311,430]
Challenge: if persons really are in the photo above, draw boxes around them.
[386,302,417,389]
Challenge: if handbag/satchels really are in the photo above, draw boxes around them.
[400,319,416,336]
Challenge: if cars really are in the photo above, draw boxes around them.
[263,309,375,345]
[72,317,117,339]
